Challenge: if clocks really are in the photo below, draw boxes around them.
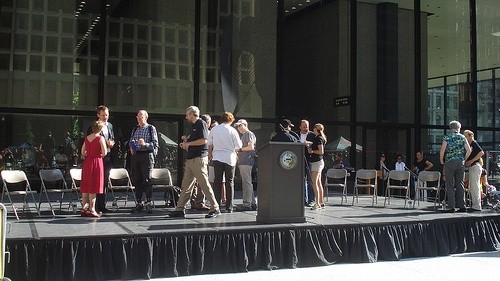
[279,150,299,170]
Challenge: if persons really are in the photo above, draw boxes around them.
[79,120,107,217]
[376,120,496,213]
[87,105,115,213]
[0,128,177,173]
[128,105,327,218]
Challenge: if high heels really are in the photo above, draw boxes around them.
[80,208,99,217]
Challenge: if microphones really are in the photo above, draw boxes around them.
[278,123,296,142]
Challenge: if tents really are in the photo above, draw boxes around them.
[324,136,362,152]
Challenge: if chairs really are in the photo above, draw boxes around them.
[383,171,411,208]
[1,170,38,221]
[150,168,177,208]
[412,171,442,210]
[465,173,487,207]
[323,168,348,205]
[352,169,378,206]
[39,169,77,217]
[68,168,83,211]
[108,168,137,211]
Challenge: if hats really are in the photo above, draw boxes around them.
[234,119,248,126]
[282,118,295,128]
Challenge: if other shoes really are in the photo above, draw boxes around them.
[457,207,467,212]
[310,203,325,210]
[169,210,187,217]
[305,202,314,207]
[442,208,455,212]
[205,209,221,218]
[467,208,481,211]
[228,209,234,213]
[95,205,110,213]
[131,204,152,213]
[235,204,257,211]
[192,203,210,209]
